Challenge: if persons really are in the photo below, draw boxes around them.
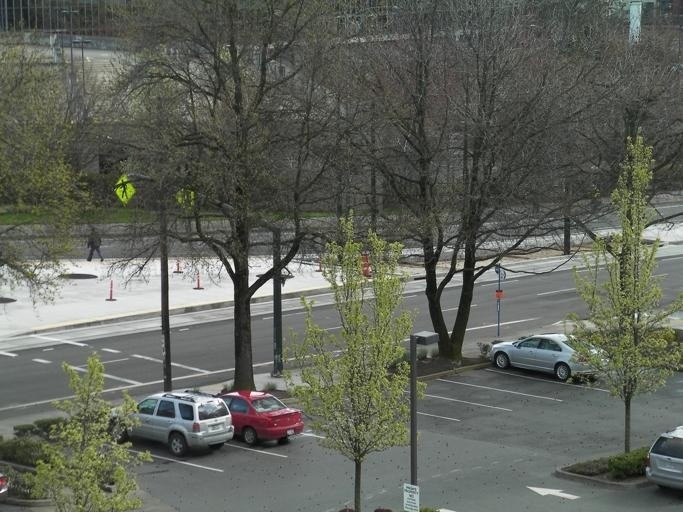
[86,227,104,262]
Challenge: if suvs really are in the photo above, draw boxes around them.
[102,390,235,458]
[643,424,682,493]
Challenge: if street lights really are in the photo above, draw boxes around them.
[408,329,439,511]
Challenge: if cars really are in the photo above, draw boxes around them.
[215,389,304,445]
[0,472,10,501]
[485,332,609,382]
